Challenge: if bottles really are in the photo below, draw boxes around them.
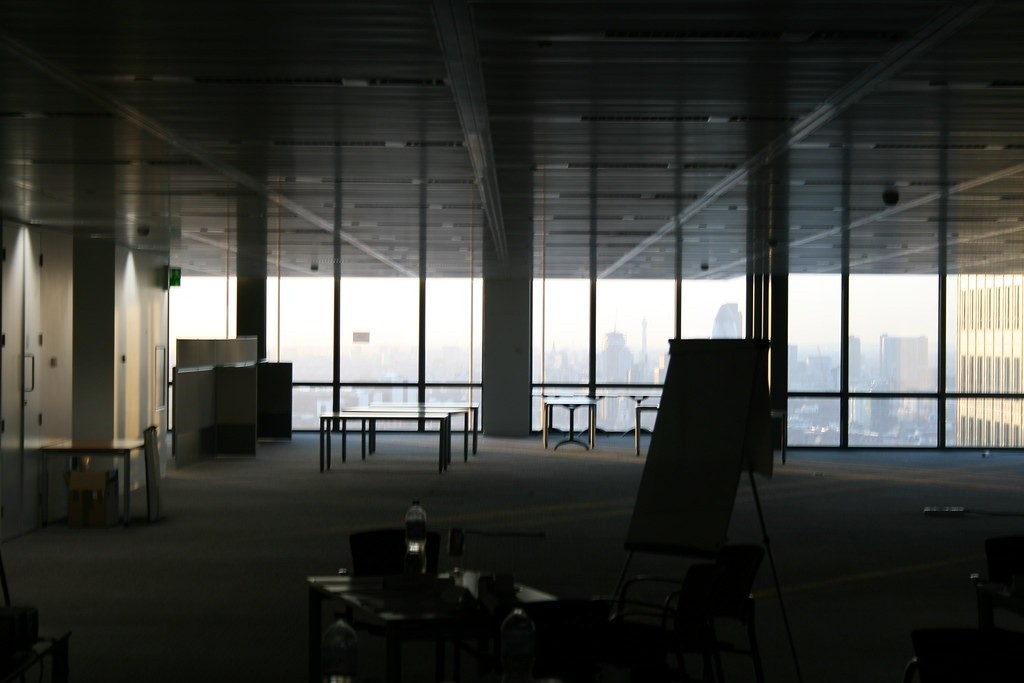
[501,607,535,683]
[322,610,358,683]
[404,500,425,574]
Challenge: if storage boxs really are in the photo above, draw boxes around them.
[44,468,119,527]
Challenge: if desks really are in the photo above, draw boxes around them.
[302,574,558,683]
[319,413,449,476]
[635,404,660,457]
[341,407,470,464]
[36,437,145,528]
[367,404,480,457]
[542,398,597,452]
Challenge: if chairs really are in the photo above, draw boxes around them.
[904,531,1024,683]
[567,540,765,683]
